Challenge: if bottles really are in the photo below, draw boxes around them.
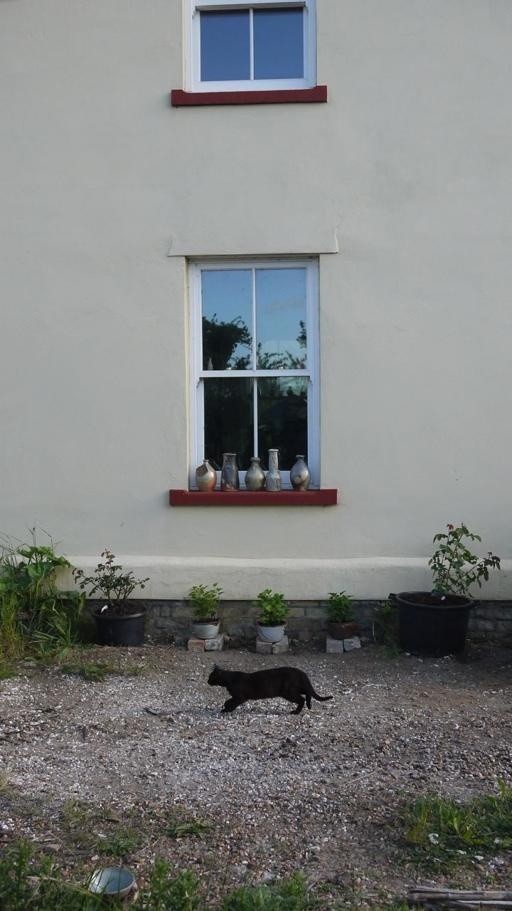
[195,448,311,492]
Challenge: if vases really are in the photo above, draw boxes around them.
[195,449,310,492]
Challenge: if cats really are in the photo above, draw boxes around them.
[207,665,333,714]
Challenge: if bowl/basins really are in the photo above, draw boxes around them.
[89,868,135,901]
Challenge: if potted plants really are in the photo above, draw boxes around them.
[72,545,150,647]
[326,590,357,639]
[255,588,289,642]
[391,522,501,654]
[188,583,222,638]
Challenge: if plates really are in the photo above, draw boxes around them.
[110,882,139,909]
